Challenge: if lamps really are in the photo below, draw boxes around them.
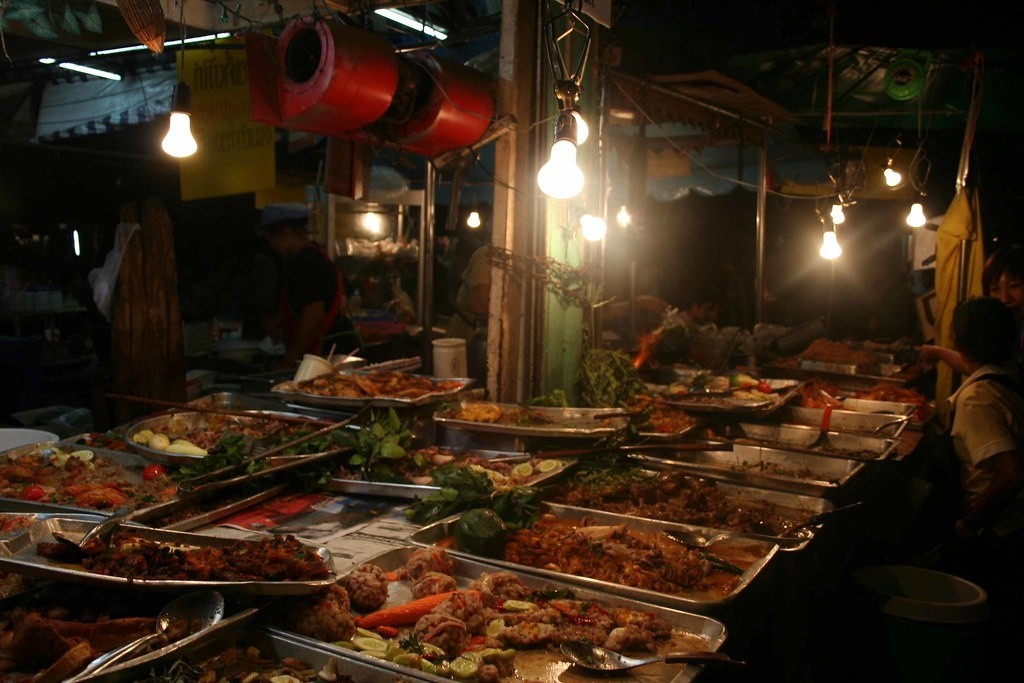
[39,57,121,82]
[89,31,231,56]
[374,6,449,41]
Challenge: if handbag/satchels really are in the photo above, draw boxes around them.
[923,432,962,514]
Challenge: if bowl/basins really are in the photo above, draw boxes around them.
[0,428,60,452]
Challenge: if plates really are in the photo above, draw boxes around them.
[272,370,477,406]
[433,400,629,437]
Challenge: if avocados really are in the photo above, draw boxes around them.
[453,508,508,559]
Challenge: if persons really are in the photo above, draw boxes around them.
[446,212,489,376]
[0,264,38,316]
[915,295,1024,609]
[41,272,116,398]
[255,202,367,365]
[649,276,720,365]
[980,244,1024,362]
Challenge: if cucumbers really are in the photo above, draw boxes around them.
[332,598,531,679]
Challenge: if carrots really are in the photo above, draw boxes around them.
[355,587,482,636]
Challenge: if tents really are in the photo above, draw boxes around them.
[586,64,829,374]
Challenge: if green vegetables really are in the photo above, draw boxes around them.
[164,409,550,530]
[526,349,657,488]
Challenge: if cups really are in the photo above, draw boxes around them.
[294,354,336,383]
[432,338,466,378]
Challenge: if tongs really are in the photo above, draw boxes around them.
[176,404,374,497]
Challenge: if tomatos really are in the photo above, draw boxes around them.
[757,381,772,393]
[19,485,46,500]
[142,464,164,480]
